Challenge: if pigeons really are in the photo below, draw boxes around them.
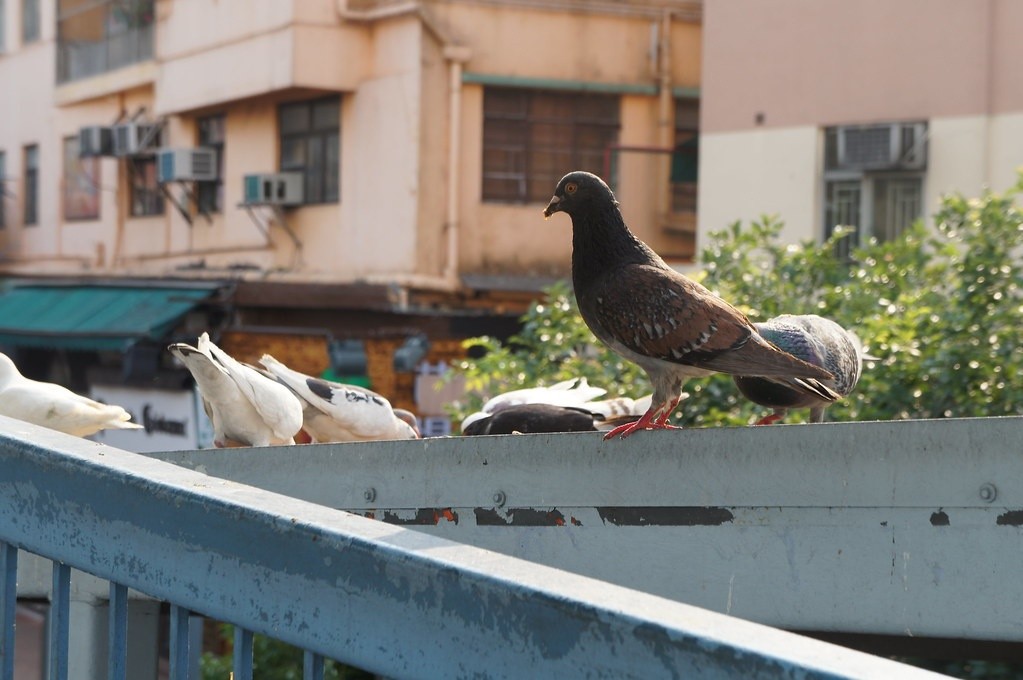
[168,332,422,451]
[0,354,145,439]
[461,171,867,442]
